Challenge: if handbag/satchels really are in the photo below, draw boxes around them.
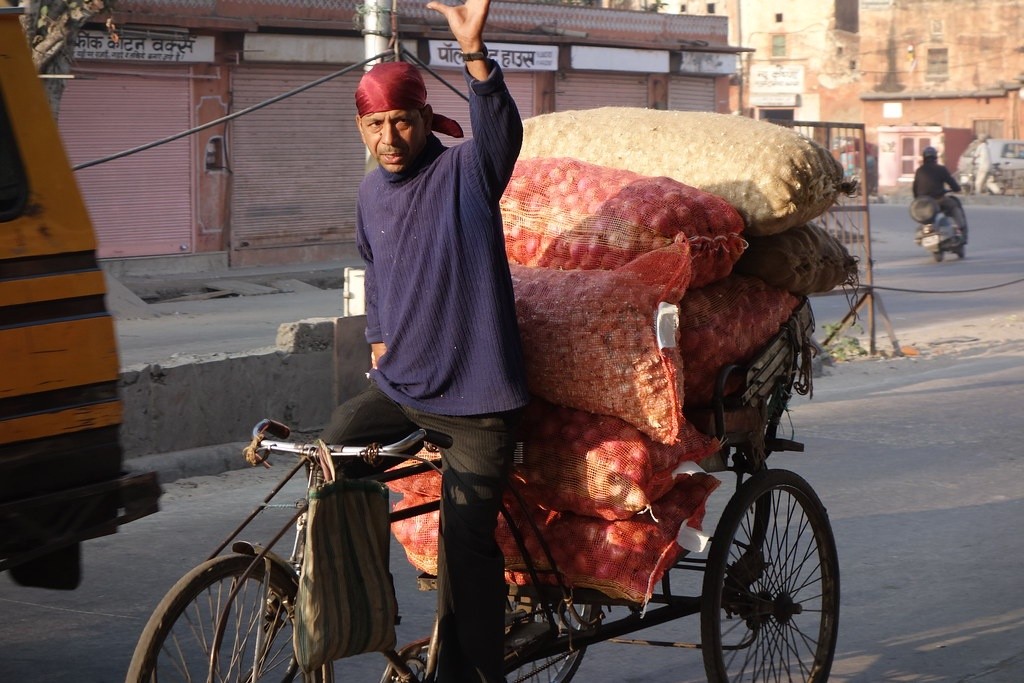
[292,439,396,675]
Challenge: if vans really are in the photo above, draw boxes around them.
[0,2,168,594]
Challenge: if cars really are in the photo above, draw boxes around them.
[959,139,1024,176]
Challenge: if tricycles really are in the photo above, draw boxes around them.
[123,295,843,683]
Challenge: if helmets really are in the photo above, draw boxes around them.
[924,147,938,158]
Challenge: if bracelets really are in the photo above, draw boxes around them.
[460,43,489,62]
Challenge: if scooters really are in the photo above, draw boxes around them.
[912,188,969,263]
[959,151,1005,195]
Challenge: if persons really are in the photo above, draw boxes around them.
[302,0,523,683]
[970,134,1005,195]
[910,147,968,241]
